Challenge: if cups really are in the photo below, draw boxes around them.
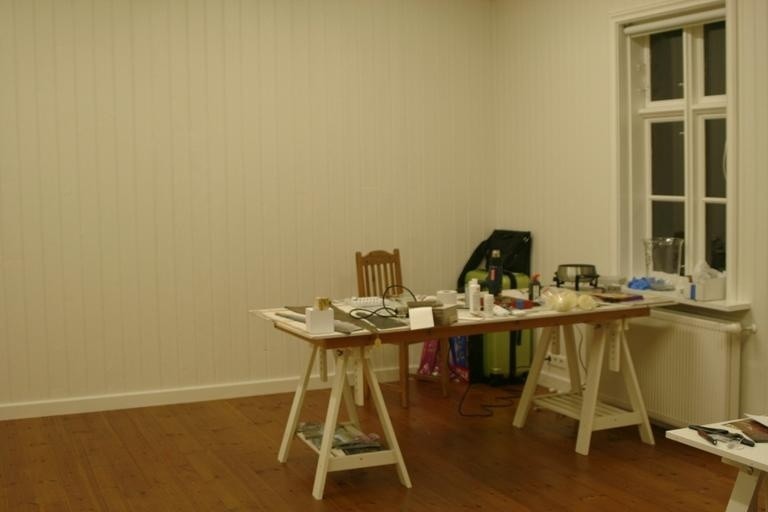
[639,235,686,291]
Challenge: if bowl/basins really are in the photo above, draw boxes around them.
[557,263,598,283]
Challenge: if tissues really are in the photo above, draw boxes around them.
[305,297,334,334]
[437,289,457,304]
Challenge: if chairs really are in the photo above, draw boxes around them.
[355,248,451,408]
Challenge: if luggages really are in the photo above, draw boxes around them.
[464,267,532,387]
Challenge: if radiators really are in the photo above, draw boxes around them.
[582,307,743,430]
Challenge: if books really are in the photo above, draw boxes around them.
[301,423,384,459]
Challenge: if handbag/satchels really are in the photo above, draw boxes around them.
[484,229,530,275]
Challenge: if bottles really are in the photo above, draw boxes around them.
[463,277,495,318]
[487,249,504,296]
[528,274,543,300]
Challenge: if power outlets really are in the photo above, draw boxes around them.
[547,354,567,369]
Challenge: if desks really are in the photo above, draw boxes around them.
[665,417,768,512]
[247,285,655,502]
